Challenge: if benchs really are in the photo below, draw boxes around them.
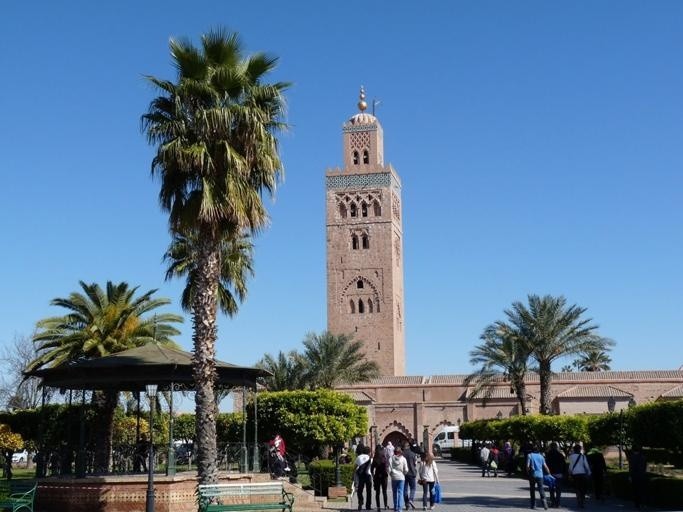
[0,478,38,512]
[195,480,295,512]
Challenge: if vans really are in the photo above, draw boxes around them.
[432,426,494,452]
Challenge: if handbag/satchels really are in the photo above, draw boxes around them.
[491,461,498,468]
[434,484,441,503]
[545,475,555,486]
[357,459,370,477]
[419,479,427,485]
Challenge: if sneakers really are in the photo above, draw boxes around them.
[530,498,560,509]
[424,505,436,511]
[358,500,389,511]
[406,500,416,510]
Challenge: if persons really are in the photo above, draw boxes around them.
[479,442,491,477]
[585,446,607,501]
[525,445,552,511]
[339,439,440,512]
[565,445,591,509]
[267,432,286,479]
[544,442,564,508]
[502,441,513,477]
[627,444,648,511]
[488,444,500,477]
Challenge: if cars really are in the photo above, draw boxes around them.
[6,449,36,463]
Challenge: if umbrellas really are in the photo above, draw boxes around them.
[543,474,556,489]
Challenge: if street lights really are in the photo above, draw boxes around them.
[145,385,158,512]
